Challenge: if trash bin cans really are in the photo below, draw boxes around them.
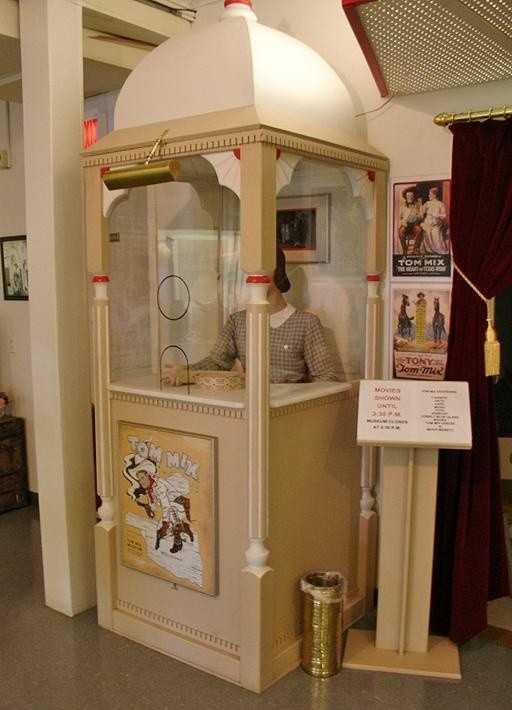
[300,571,345,678]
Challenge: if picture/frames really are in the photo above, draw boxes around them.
[117,420,219,598]
[0,234,28,301]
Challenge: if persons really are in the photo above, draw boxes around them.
[399,186,448,255]
[411,292,427,344]
[160,241,338,387]
[136,470,193,553]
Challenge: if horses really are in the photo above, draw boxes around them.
[397,294,414,338]
[433,296,447,343]
